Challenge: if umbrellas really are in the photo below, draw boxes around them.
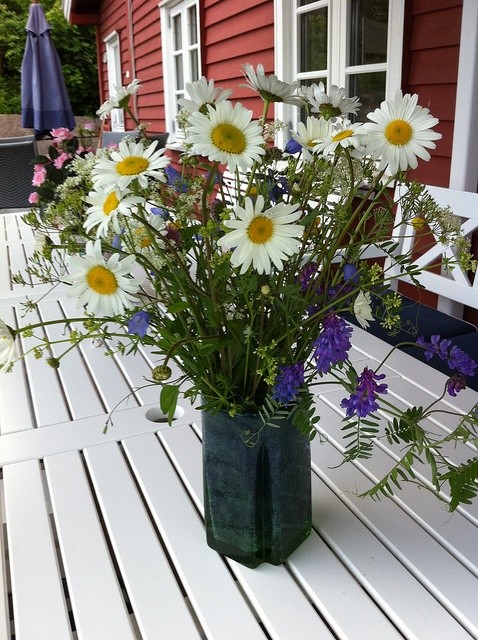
[19,2,75,138]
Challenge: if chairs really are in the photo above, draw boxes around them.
[299,181,477,392]
[0,133,39,212]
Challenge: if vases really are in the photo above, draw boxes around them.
[199,388,313,569]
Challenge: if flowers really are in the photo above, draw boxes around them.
[0,60,478,513]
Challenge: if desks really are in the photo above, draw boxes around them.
[0,210,476,640]
